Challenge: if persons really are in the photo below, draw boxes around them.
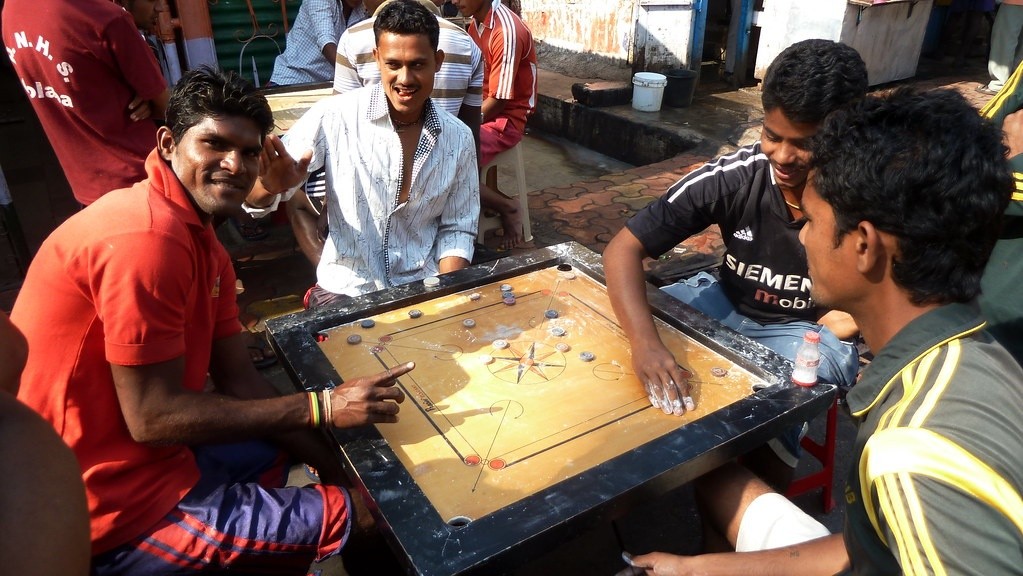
[920,0,1023,95]
[241,0,536,309]
[10,64,416,576]
[613,85,1023,576]
[602,39,868,497]
[0,314,92,576]
[975,59,1023,371]
[0,0,280,370]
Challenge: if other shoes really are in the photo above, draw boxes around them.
[975,84,998,96]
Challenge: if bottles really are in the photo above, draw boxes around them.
[791,331,820,387]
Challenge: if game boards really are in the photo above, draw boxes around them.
[264,239,838,576]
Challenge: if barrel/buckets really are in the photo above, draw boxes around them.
[632,72,668,112]
[663,69,699,108]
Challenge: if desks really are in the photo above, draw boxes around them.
[256,80,333,139]
[263,239,837,575]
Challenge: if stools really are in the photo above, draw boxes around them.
[784,401,841,513]
[476,140,534,246]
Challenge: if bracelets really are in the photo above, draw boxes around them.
[307,390,333,427]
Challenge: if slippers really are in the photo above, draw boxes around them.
[468,243,511,264]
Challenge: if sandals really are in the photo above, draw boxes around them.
[247,339,278,368]
[304,462,320,483]
[235,218,268,240]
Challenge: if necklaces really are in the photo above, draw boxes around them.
[784,199,800,210]
[390,107,425,135]
